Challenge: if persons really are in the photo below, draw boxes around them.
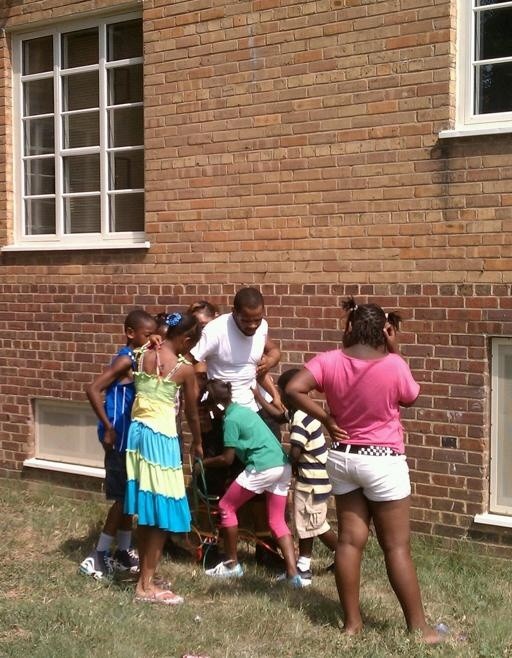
[285,293,445,644]
[78,286,337,604]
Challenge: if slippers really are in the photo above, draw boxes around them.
[135,588,183,605]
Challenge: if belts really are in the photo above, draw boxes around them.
[329,440,397,456]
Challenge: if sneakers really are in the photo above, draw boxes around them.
[79,551,114,585]
[115,549,140,572]
[325,563,335,574]
[206,561,244,577]
[277,568,312,589]
